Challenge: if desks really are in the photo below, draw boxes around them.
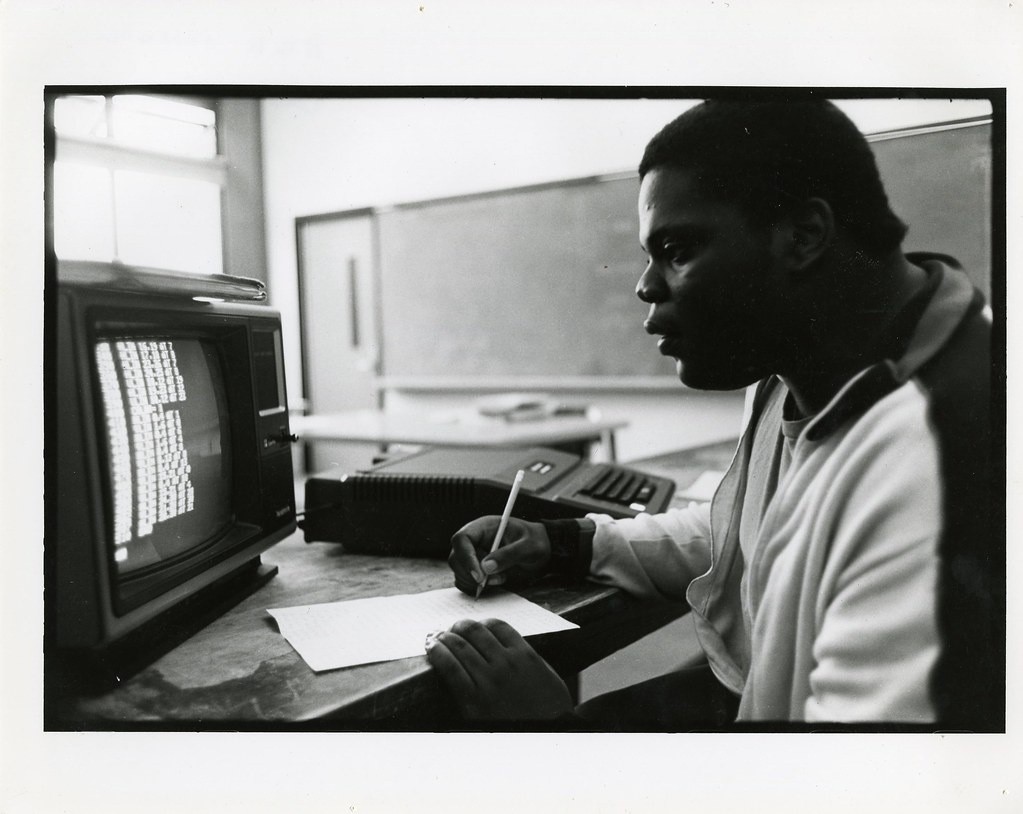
[44,438,739,732]
[291,410,630,463]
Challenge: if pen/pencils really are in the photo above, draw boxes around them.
[475,467,526,601]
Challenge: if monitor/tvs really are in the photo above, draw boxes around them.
[53,285,297,684]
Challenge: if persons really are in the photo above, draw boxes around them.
[424,97,991,723]
